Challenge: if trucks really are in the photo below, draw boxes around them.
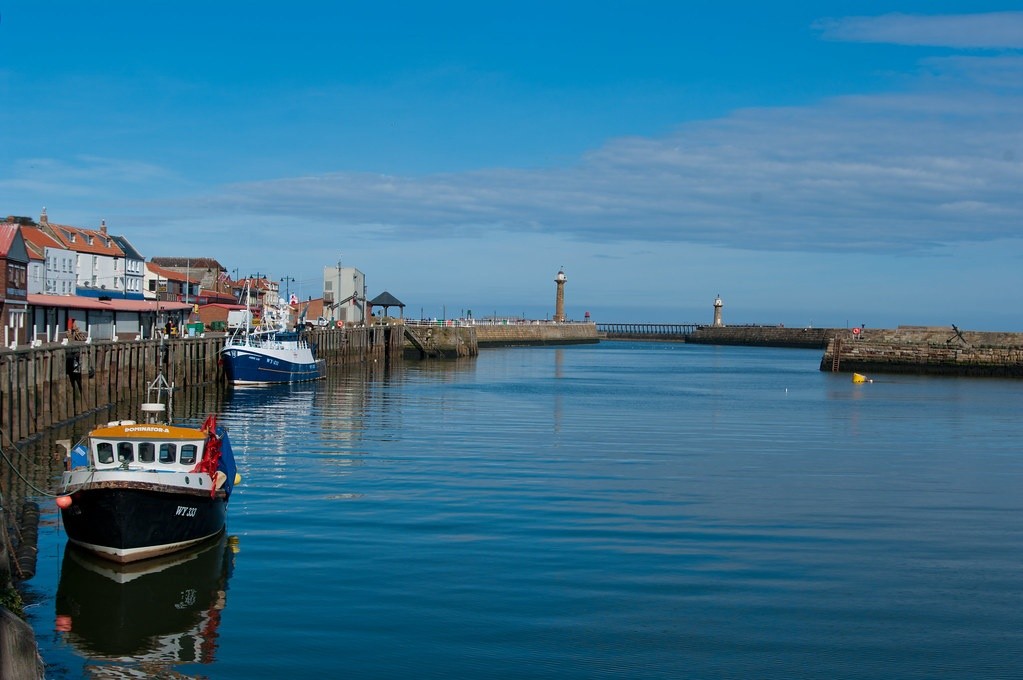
[306,316,330,328]
[228,310,253,334]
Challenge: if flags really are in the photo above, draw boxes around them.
[219,273,231,283]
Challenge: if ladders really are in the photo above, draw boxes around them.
[832,339,841,372]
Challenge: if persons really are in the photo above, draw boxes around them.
[165,320,172,339]
[71,318,81,340]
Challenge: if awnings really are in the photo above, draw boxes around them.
[26,293,193,311]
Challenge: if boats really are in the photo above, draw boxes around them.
[221,280,327,385]
[54,532,241,680]
[220,378,327,488]
[54,327,241,564]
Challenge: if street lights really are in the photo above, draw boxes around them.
[250,272,267,308]
[114,253,126,298]
[335,260,344,321]
[207,266,228,303]
[233,267,239,286]
[280,275,295,302]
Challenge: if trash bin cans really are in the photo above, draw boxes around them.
[186,321,205,337]
[211,321,228,330]
